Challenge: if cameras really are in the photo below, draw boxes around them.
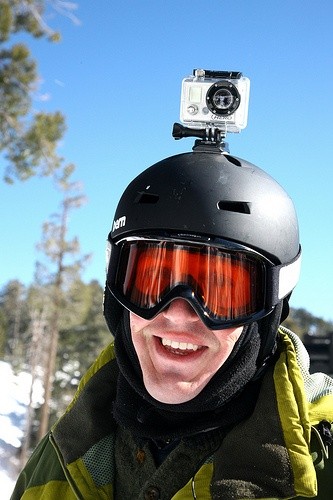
[180,68,250,133]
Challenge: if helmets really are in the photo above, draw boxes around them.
[104,66,302,331]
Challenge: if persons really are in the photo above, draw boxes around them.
[9,148,333,500]
